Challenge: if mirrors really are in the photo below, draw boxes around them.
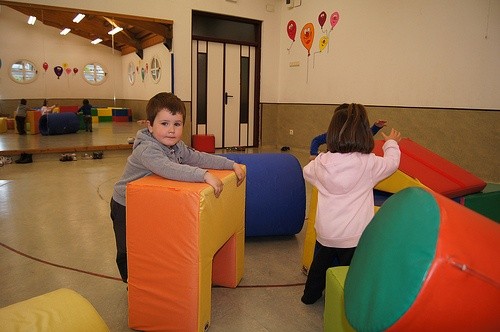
[0,0,176,153]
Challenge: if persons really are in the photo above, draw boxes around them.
[76,99,92,132]
[15,99,36,135]
[40,99,55,116]
[309,120,387,160]
[301,102,402,305]
[110,92,246,293]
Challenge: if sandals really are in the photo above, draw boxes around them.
[80,152,93,159]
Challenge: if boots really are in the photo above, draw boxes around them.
[15,153,32,163]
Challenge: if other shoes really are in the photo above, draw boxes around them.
[59,153,77,161]
[97,153,103,158]
[93,152,98,159]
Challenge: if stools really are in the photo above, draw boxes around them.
[193,134,216,153]
